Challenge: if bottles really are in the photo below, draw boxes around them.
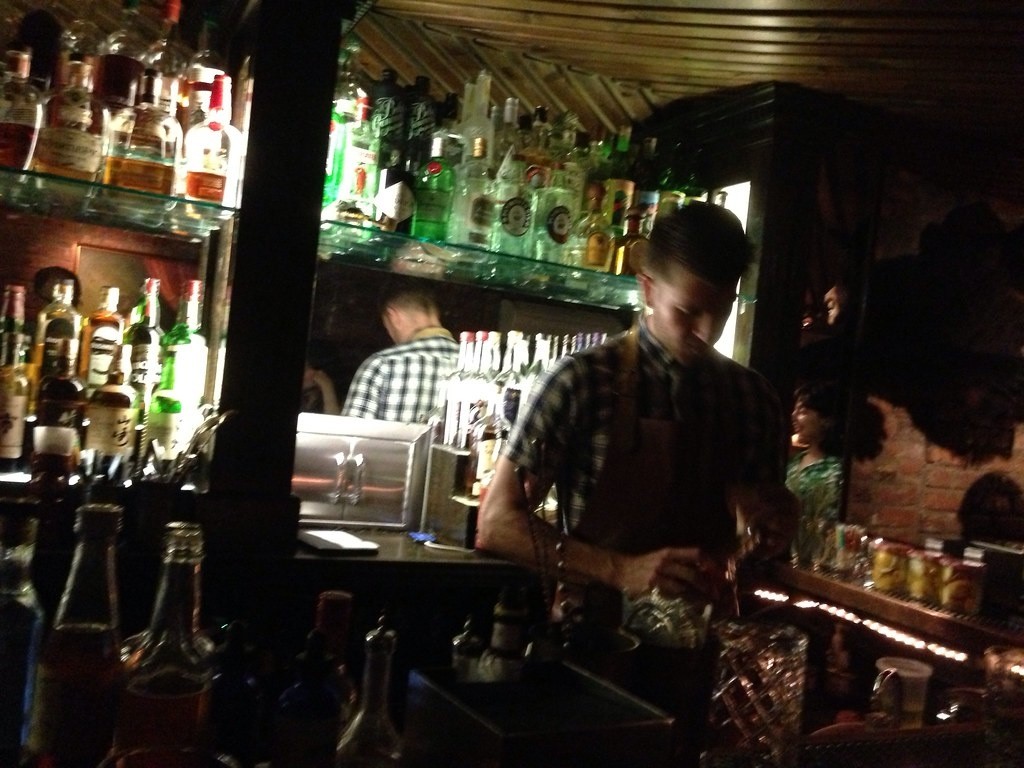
[33,280,87,477]
[0,286,32,473]
[81,286,138,479]
[263,589,355,768]
[432,332,612,483]
[0,0,258,241]
[25,503,123,768]
[328,616,414,768]
[158,280,208,460]
[317,37,706,311]
[117,522,214,767]
[0,516,47,768]
[125,275,165,432]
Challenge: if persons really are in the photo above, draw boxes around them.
[784,382,888,534]
[299,339,338,416]
[474,202,801,626]
[36,266,82,307]
[339,279,495,427]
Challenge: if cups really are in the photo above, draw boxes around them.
[868,657,932,730]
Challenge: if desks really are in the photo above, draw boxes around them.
[400,649,678,768]
[281,523,521,611]
